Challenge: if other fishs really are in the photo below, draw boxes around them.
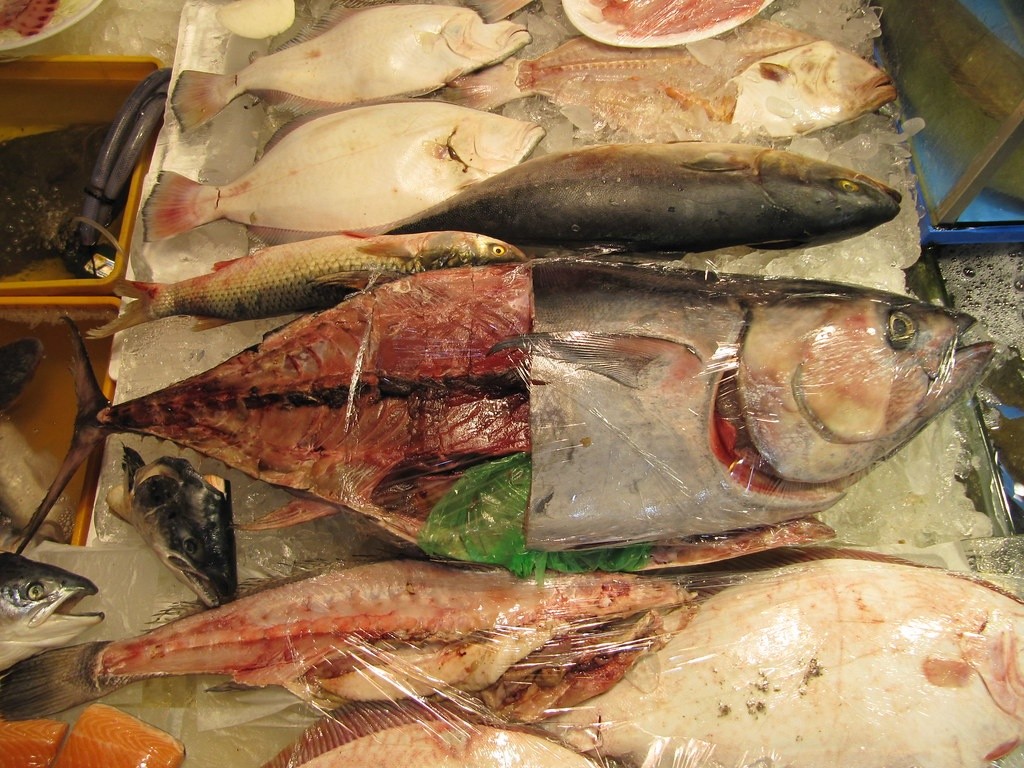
[0,456,1024,767]
[16,232,996,573]
[141,1,902,255]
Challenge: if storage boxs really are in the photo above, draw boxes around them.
[0,54,164,295]
[0,294,122,547]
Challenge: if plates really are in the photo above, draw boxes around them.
[0,0,108,52]
[562,0,773,49]
